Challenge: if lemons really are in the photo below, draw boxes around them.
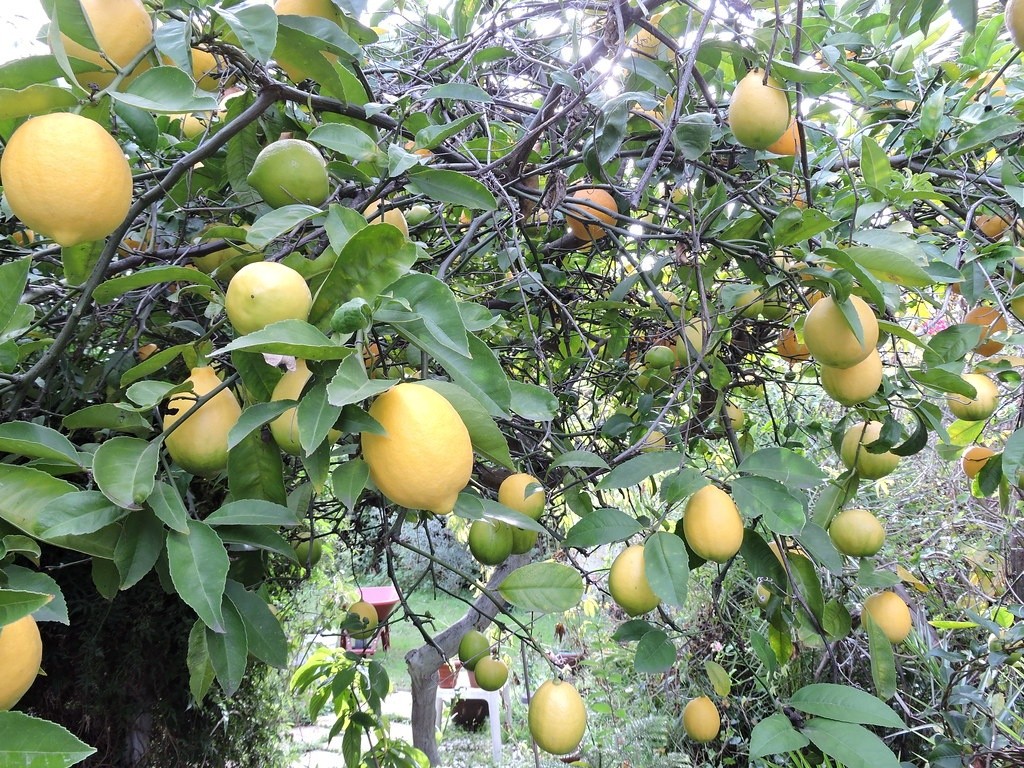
[0,0,1024,768]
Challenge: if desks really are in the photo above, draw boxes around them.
[436,680,513,765]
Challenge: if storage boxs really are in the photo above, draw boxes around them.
[440,659,478,687]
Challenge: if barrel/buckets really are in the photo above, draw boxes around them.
[439,662,461,689]
[466,670,479,688]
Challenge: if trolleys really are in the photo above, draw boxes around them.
[339,579,403,658]
